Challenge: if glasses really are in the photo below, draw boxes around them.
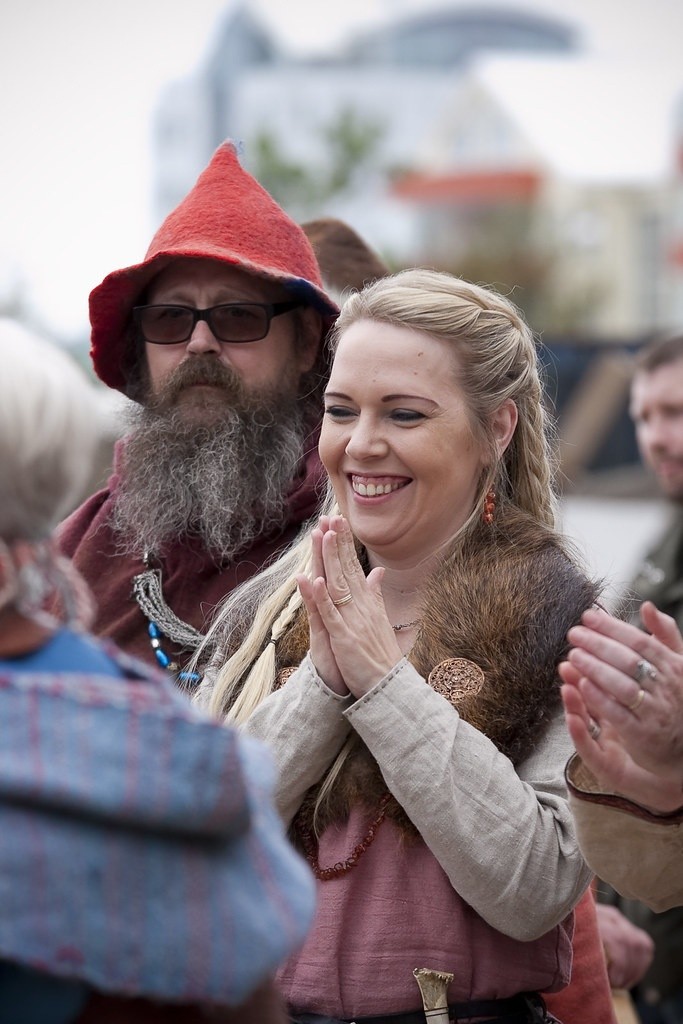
[132,300,299,345]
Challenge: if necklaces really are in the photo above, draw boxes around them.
[305,795,392,882]
[135,547,206,685]
[391,617,421,631]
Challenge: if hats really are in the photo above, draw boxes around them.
[88,143,341,408]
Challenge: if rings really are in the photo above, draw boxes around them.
[632,660,657,682]
[335,594,353,607]
[629,690,645,710]
[592,727,601,740]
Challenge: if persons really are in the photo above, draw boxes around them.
[301,221,391,295]
[558,602,682,913]
[52,140,654,987]
[619,334,683,629]
[195,270,592,1024]
[1,451,317,1024]
